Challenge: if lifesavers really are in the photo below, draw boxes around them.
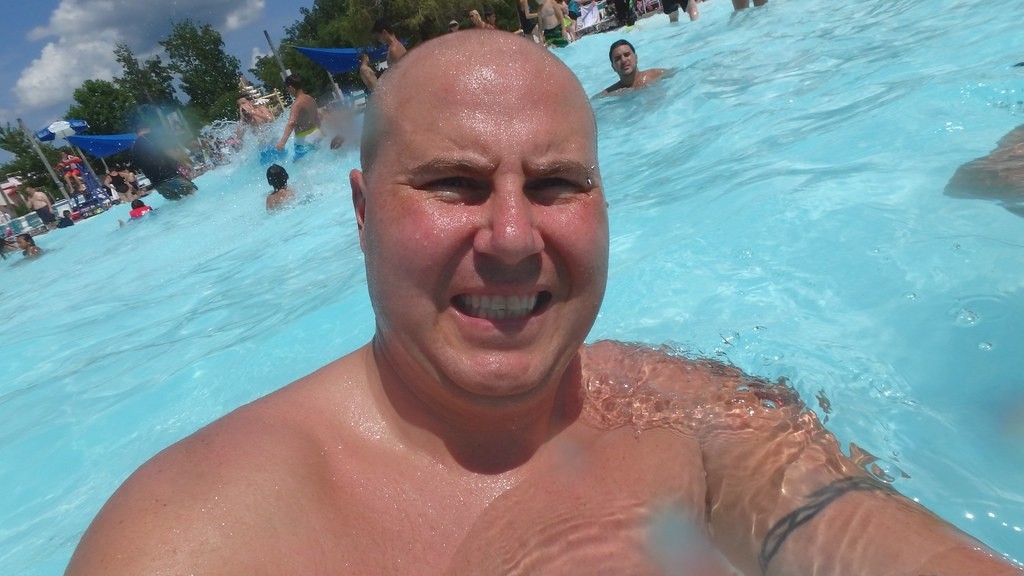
[54,158,83,171]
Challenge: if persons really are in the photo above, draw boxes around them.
[54,150,85,193]
[449,20,459,32]
[359,53,379,108]
[517,0,772,49]
[236,93,288,165]
[469,10,498,29]
[1,210,12,223]
[178,134,245,181]
[118,199,153,228]
[26,187,59,231]
[64,29,1024,576]
[372,18,408,68]
[943,124,1024,219]
[16,233,43,260]
[103,166,141,202]
[0,237,19,260]
[133,123,199,200]
[63,210,77,224]
[276,74,329,163]
[266,163,324,216]
[590,39,679,112]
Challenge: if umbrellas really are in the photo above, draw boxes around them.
[36,118,90,157]
